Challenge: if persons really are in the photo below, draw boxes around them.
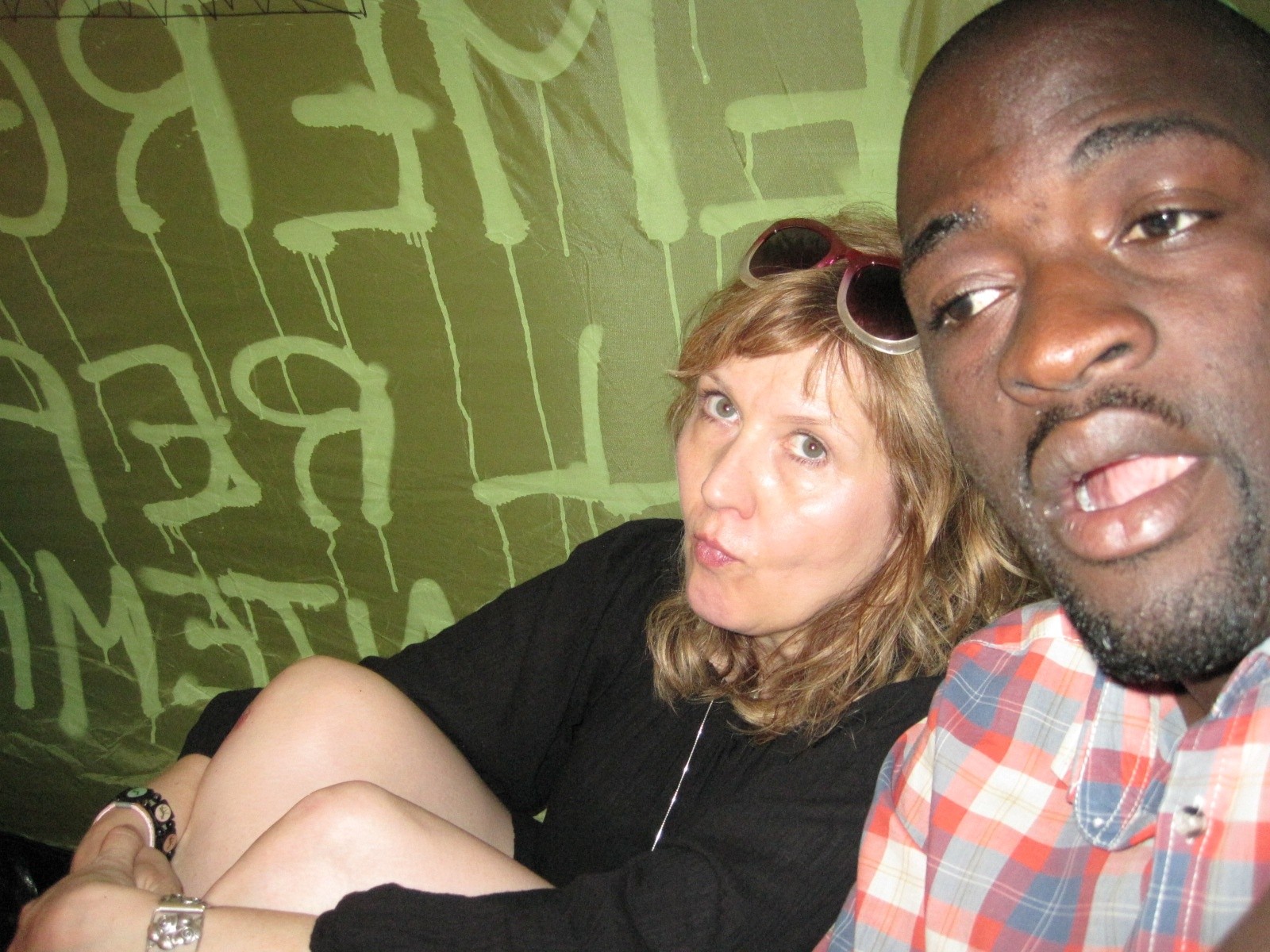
[4,214,1041,952]
[814,1,1269,950]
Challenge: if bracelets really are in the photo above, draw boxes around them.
[145,892,209,952]
[91,786,178,863]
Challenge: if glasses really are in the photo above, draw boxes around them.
[739,219,922,356]
